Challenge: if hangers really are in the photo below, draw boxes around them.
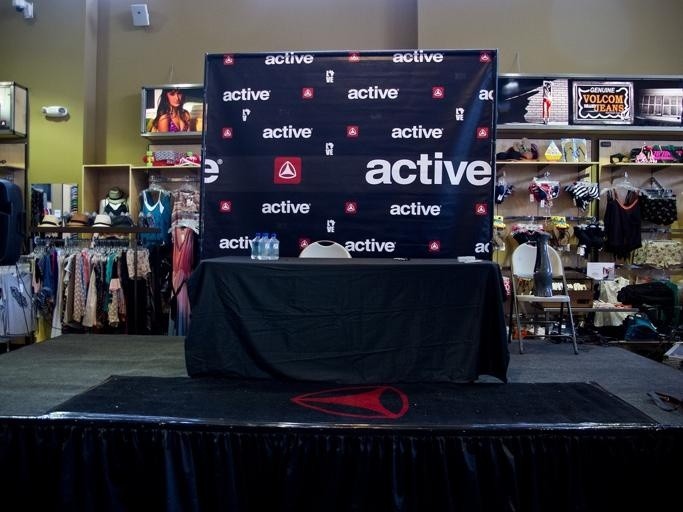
[32,176,197,252]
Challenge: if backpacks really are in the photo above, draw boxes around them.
[617,282,674,308]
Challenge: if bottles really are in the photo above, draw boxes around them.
[269,234,280,260]
[257,233,270,261]
[251,233,260,259]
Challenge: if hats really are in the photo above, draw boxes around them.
[38,215,133,227]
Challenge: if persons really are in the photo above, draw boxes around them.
[153,89,191,132]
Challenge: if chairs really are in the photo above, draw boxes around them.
[510,240,581,355]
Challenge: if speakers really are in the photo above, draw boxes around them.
[131,4,149,27]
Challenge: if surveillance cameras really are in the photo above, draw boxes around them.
[12,0,33,19]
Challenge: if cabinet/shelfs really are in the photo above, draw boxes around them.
[492,74,683,349]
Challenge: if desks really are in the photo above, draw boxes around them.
[183,256,512,386]
